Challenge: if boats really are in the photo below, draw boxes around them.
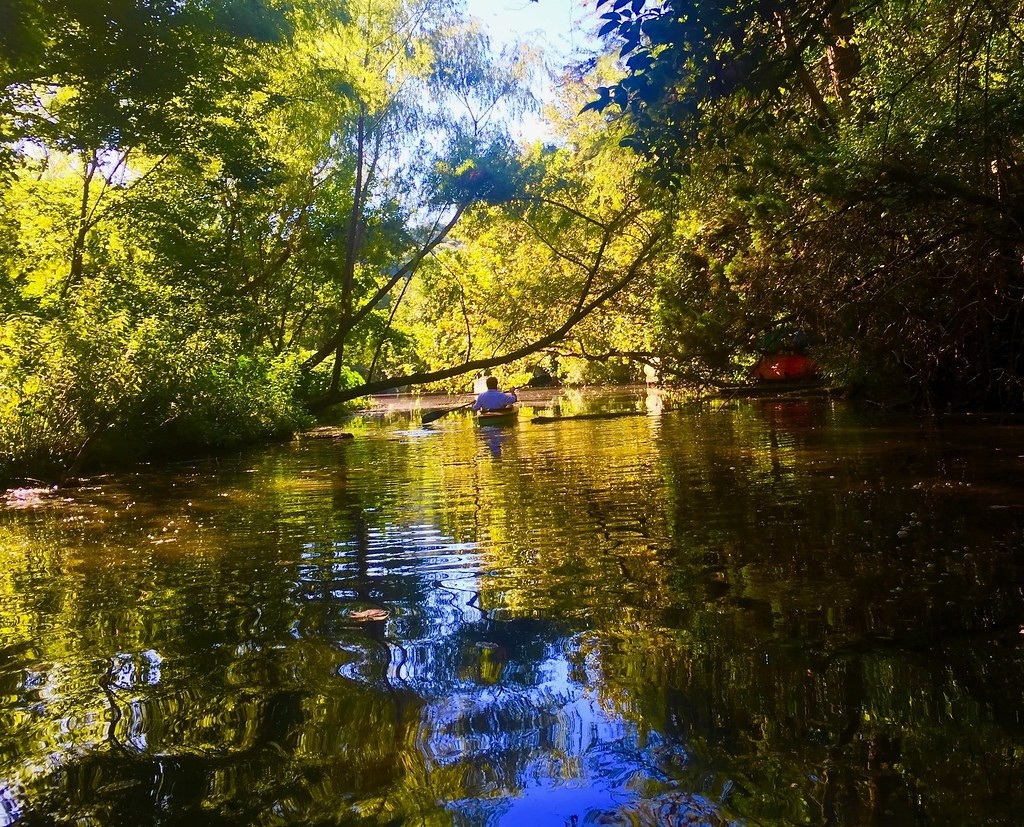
[473,405,519,426]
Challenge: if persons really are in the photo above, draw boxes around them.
[472,378,517,411]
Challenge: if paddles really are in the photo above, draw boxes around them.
[421,386,523,423]
